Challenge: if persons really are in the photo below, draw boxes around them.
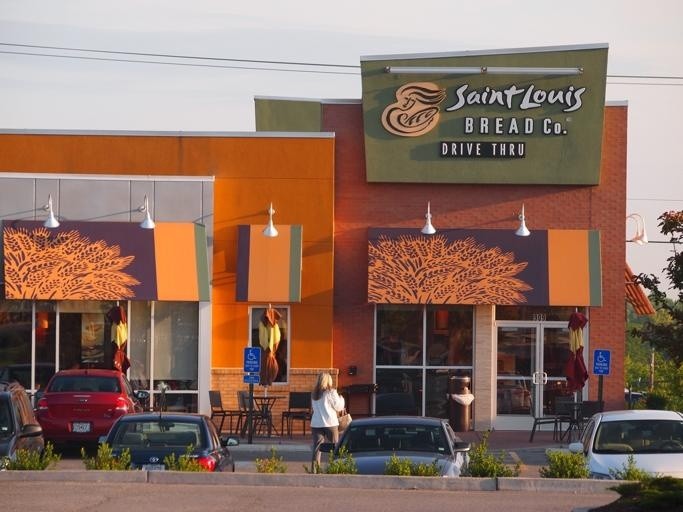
[598,352,605,361]
[249,349,256,360]
[308,372,345,473]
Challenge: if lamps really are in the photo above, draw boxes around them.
[41,193,58,229]
[33,309,48,330]
[430,304,449,337]
[626,212,645,246]
[515,199,533,239]
[420,197,437,236]
[625,207,649,246]
[260,199,278,238]
[137,193,157,232]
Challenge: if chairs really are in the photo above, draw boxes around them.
[201,384,319,445]
[517,390,608,449]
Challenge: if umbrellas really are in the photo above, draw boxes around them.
[565,307,588,443]
[258,302,282,438]
[106,300,131,374]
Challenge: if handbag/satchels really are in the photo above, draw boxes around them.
[338,408,353,433]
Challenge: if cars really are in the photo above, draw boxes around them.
[496,371,532,413]
[34,369,148,447]
[624,388,641,402]
[317,414,472,477]
[0,379,48,469]
[375,371,422,415]
[95,411,241,472]
[568,408,682,484]
[0,363,63,394]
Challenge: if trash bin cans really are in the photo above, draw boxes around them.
[447,377,471,432]
